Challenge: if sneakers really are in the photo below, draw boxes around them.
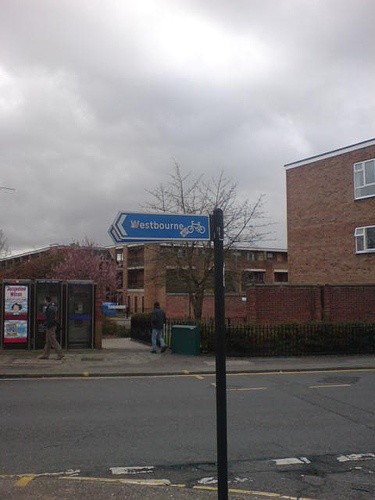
[161,345,168,353]
[150,350,157,353]
[56,356,65,360]
[38,355,49,359]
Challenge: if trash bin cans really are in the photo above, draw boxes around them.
[171,326,200,353]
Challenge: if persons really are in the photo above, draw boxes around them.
[37,296,65,360]
[150,302,168,353]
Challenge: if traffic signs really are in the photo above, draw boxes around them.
[106,222,174,243]
[112,210,210,241]
